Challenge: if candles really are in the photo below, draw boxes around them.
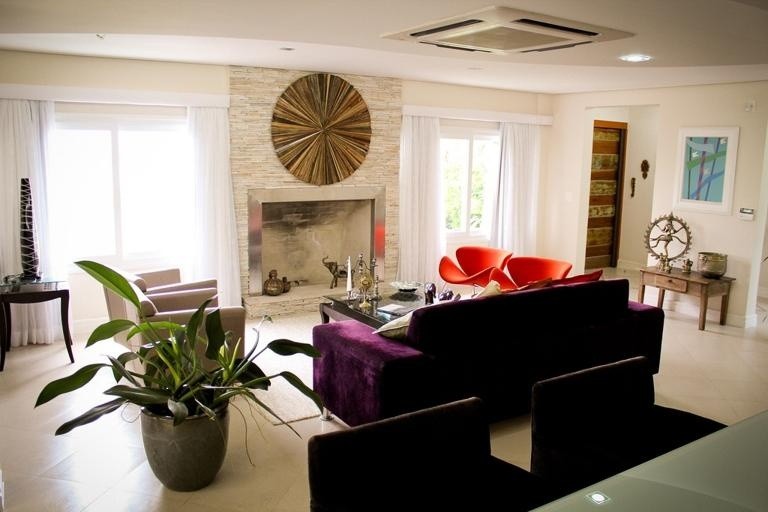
[347,256,353,300]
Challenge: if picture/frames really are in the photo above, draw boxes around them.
[672,126,740,215]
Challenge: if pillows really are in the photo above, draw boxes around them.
[371,300,454,340]
[519,270,603,291]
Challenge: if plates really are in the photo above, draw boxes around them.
[389,281,424,292]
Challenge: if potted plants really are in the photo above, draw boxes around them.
[33,260,324,492]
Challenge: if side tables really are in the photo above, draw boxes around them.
[0,280,74,372]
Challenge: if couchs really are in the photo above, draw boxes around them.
[103,265,218,350]
[312,278,665,427]
[125,280,245,373]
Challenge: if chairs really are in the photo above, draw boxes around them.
[489,256,572,294]
[307,397,567,511]
[439,246,513,298]
[529,355,728,489]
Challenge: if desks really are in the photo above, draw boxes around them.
[637,266,736,330]
[525,411,768,512]
